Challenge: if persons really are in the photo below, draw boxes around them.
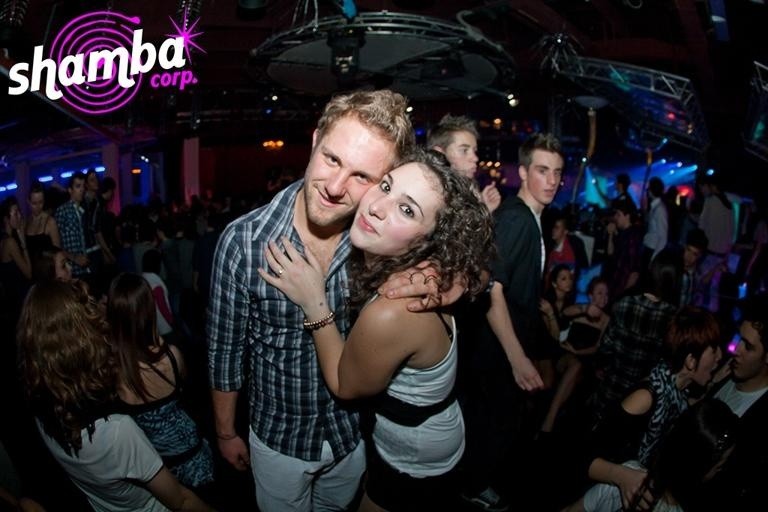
[202,85,416,511]
[0,113,767,512]
